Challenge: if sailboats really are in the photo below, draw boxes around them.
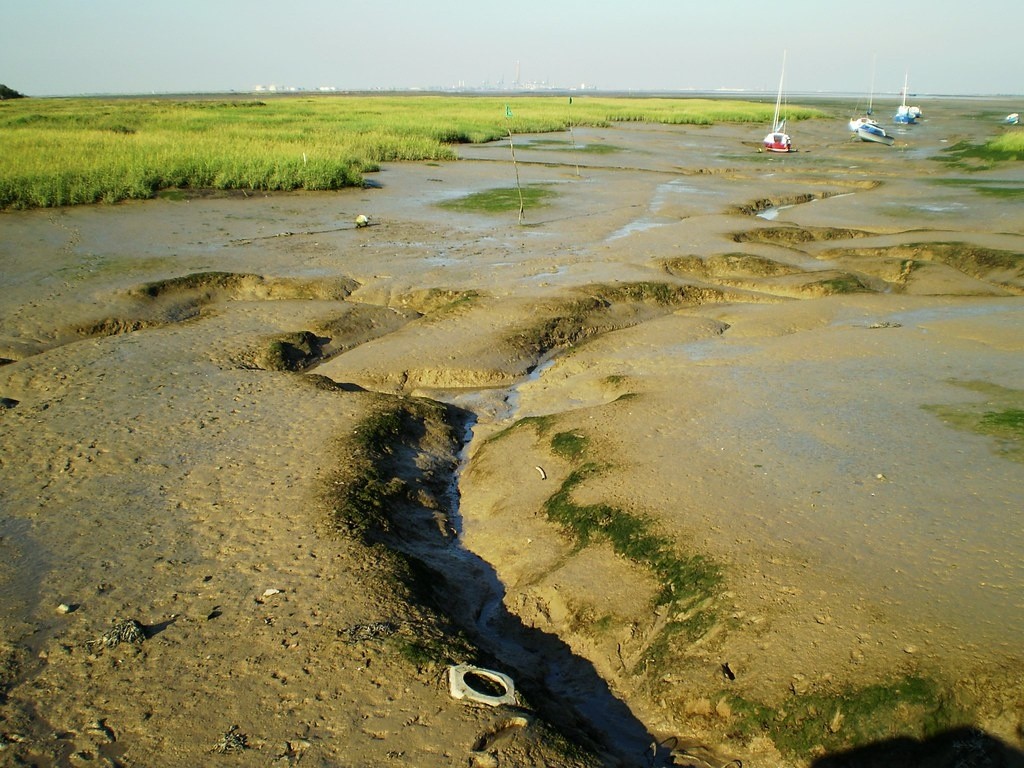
[858,65,895,146]
[849,67,879,131]
[763,49,792,153]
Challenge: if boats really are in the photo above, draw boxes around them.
[893,72,916,124]
[1006,113,1020,124]
[907,105,922,117]
